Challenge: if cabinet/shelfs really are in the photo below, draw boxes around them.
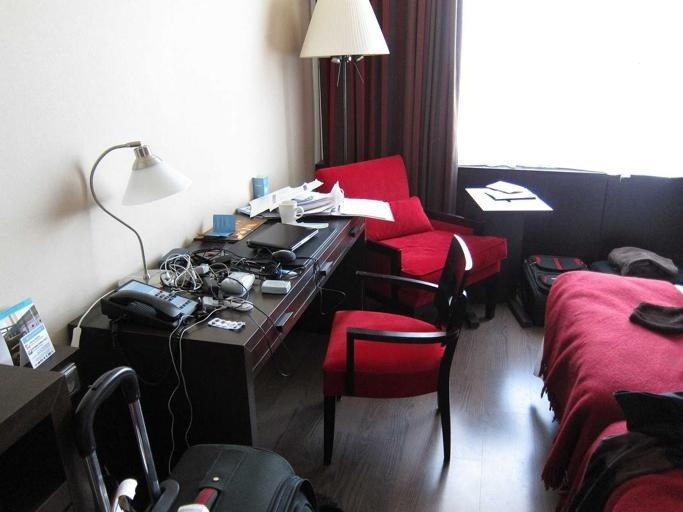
[602,175,683,260]
[0,363,97,512]
[458,165,606,304]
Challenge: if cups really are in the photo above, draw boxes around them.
[279,200,304,225]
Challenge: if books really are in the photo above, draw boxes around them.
[486,180,536,200]
[237,186,394,222]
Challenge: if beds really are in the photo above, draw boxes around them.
[559,420,683,512]
[534,270,683,491]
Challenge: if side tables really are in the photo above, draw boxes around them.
[465,187,553,302]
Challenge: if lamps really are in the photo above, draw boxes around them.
[90,141,193,285]
[299,0,390,165]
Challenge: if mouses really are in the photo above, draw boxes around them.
[272,250,296,262]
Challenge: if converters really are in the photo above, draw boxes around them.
[201,277,219,297]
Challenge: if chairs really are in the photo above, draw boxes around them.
[321,234,473,467]
[313,154,508,318]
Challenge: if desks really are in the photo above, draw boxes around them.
[69,210,366,446]
[25,342,96,411]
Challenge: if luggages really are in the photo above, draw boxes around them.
[71,365,317,512]
[520,254,588,328]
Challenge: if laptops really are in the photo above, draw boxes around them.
[245,222,318,250]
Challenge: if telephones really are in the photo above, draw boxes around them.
[100,279,198,331]
